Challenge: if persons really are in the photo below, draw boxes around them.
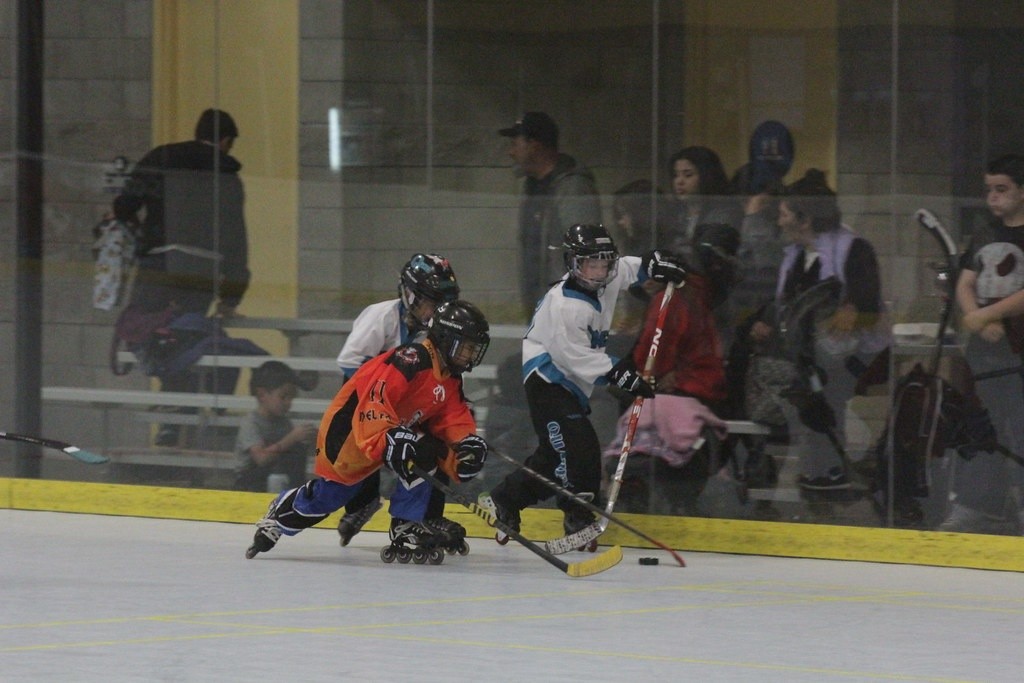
[223,361,318,494]
[485,223,687,551]
[500,113,1024,490]
[244,300,486,564]
[337,252,471,557]
[106,108,251,446]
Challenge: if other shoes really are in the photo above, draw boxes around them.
[938,505,977,532]
[796,466,851,491]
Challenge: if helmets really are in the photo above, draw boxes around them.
[428,299,491,378]
[560,221,619,293]
[400,253,462,334]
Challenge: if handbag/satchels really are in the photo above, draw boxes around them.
[739,353,803,424]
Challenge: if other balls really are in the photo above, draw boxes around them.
[638,557,659,565]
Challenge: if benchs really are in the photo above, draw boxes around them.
[32,314,967,499]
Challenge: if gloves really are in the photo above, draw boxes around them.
[648,252,687,288]
[456,435,488,483]
[605,358,657,399]
[383,425,418,479]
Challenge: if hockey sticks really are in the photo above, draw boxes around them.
[0,431,111,466]
[491,446,688,567]
[914,205,962,378]
[407,459,625,580]
[542,281,677,557]
[781,279,889,525]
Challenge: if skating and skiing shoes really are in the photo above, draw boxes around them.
[245,497,282,559]
[338,493,384,546]
[563,509,597,552]
[380,515,448,565]
[477,491,521,546]
[426,517,470,555]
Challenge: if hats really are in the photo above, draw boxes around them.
[499,111,560,147]
